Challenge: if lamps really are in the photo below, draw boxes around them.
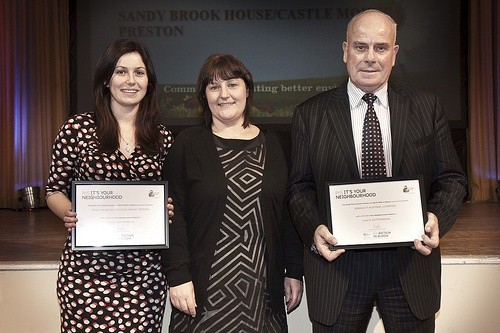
[18,186,40,210]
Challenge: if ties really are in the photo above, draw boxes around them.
[361,92,388,179]
